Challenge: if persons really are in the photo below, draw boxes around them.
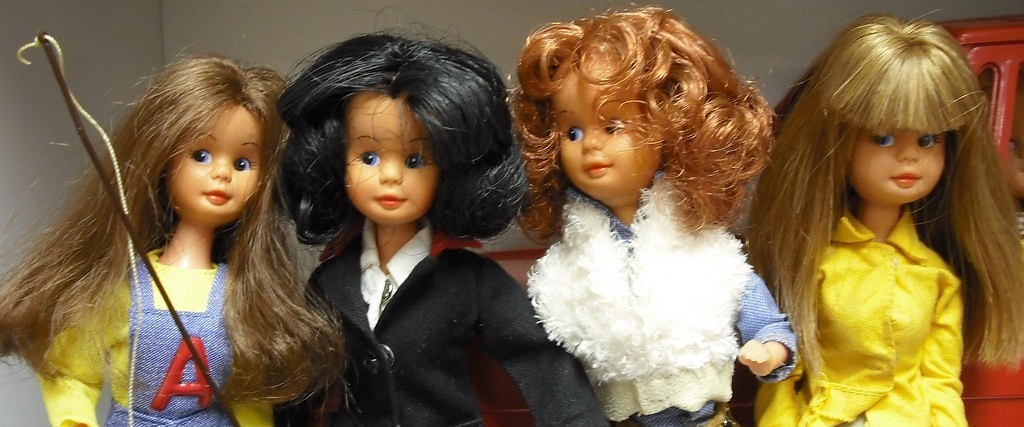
[505,6,797,427]
[274,32,610,427]
[0,55,347,427]
[737,11,1024,427]
